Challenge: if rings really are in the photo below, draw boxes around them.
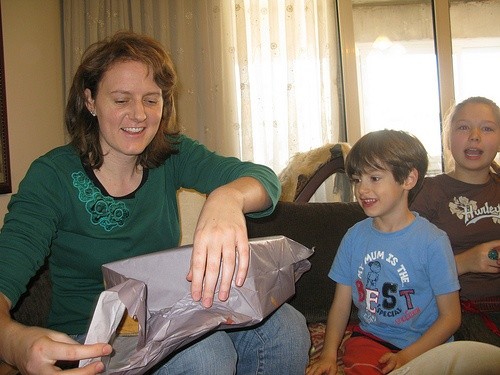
[488,248,498,260]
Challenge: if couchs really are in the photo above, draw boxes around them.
[10,202,368,375]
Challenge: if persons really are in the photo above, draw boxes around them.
[407,95,500,350]
[0,31,312,375]
[305,129,464,374]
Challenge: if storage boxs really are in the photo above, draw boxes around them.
[102,235,295,336]
